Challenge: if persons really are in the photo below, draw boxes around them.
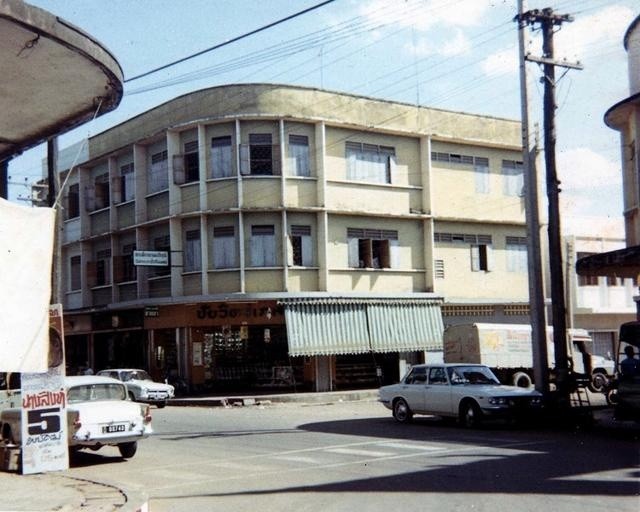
[619,346,639,379]
[82,360,94,377]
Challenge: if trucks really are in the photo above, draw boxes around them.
[445,322,615,393]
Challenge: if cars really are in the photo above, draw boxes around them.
[1,373,153,459]
[377,361,543,426]
[97,369,173,408]
